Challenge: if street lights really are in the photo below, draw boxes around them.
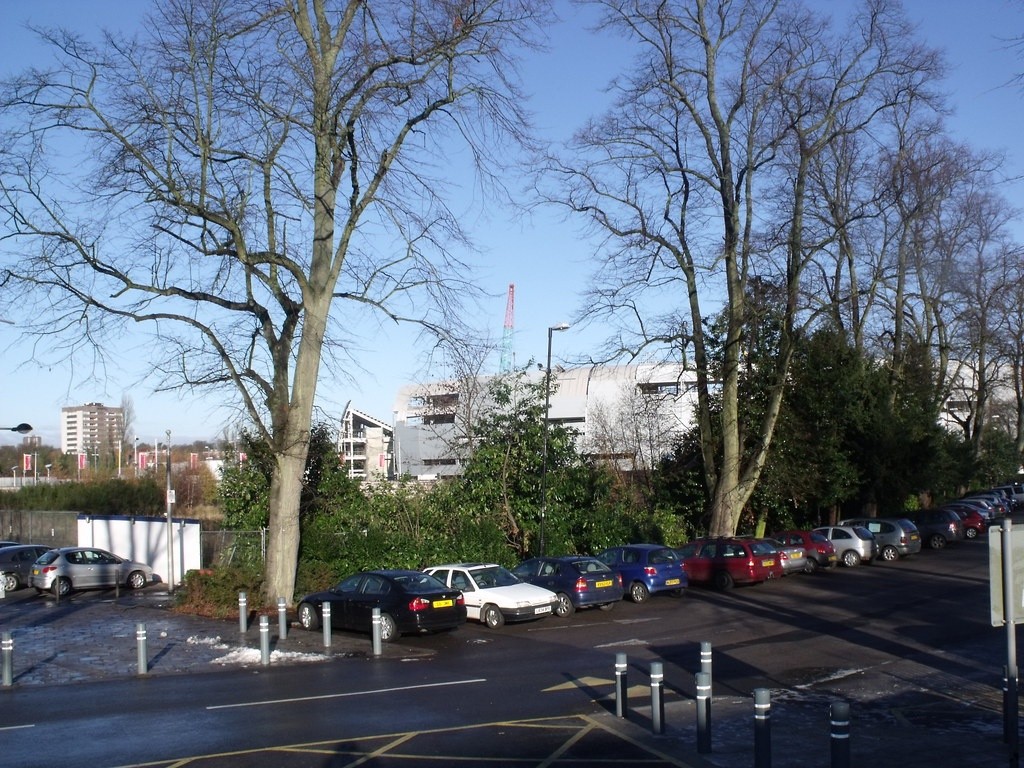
[0,422,34,435]
[542,323,568,507]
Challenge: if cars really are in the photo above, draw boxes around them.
[298,570,467,643]
[0,542,22,549]
[932,504,987,539]
[761,537,808,578]
[28,547,153,596]
[675,536,782,595]
[893,509,964,550]
[838,518,922,562]
[423,562,560,629]
[0,545,55,593]
[810,526,878,567]
[510,556,624,612]
[945,482,1024,523]
[597,544,691,604]
[772,530,836,576]
